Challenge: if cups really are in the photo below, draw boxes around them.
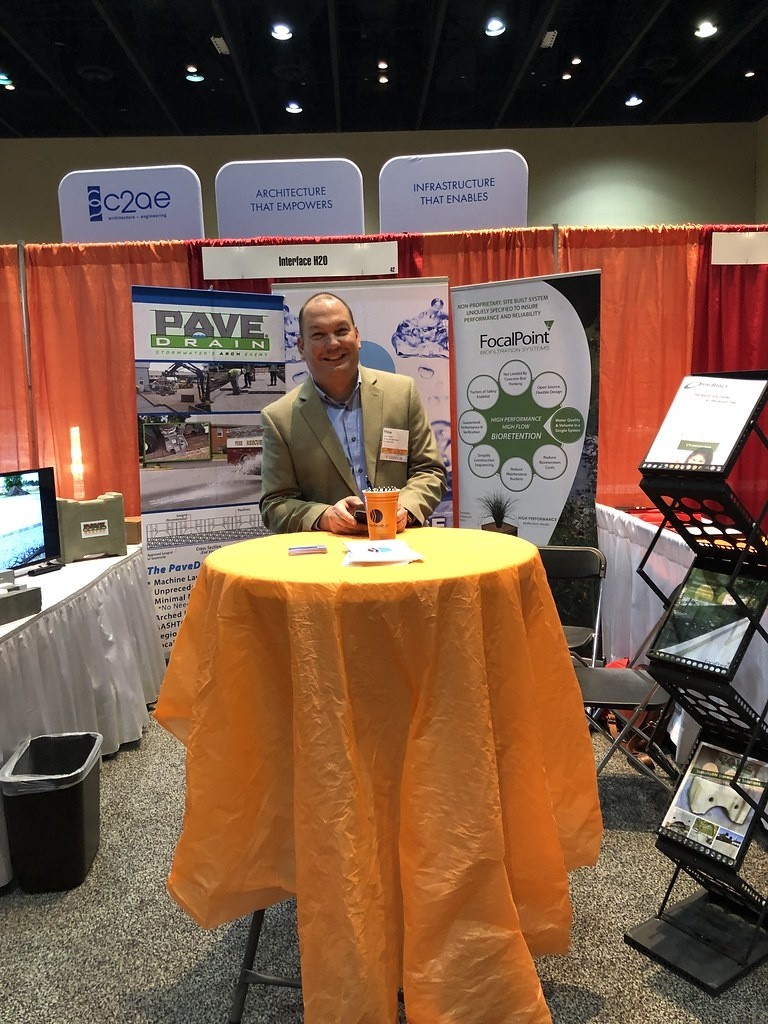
[363,487,400,541]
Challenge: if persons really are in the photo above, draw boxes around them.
[259,293,448,540]
[242,363,254,389]
[268,362,276,386]
[227,369,246,396]
[668,448,713,474]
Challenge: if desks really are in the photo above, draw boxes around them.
[0,543,165,889]
[148,525,605,1024]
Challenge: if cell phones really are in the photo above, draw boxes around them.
[354,510,368,524]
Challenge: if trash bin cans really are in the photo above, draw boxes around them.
[0,731,105,895]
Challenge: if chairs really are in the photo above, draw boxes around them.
[533,545,610,733]
[568,582,697,799]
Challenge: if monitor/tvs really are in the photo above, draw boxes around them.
[0,467,62,571]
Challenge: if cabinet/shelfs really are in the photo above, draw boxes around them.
[617,368,768,1001]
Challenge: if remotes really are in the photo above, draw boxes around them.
[28,565,61,576]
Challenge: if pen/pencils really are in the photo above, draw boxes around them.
[362,476,400,539]
